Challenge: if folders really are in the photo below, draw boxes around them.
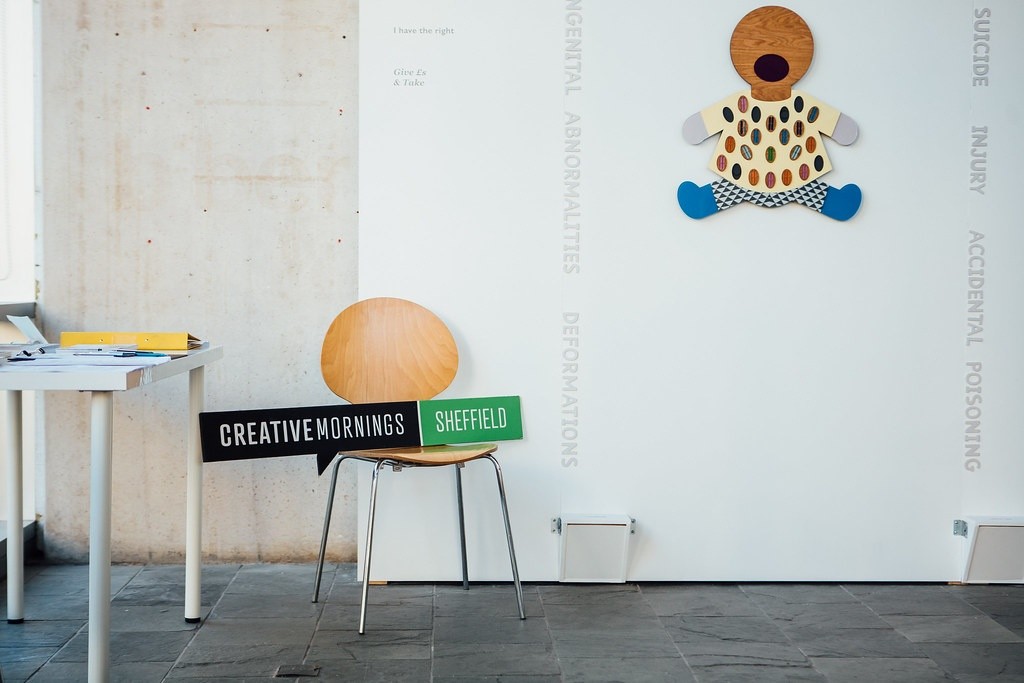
[60,332,201,351]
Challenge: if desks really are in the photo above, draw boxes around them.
[0,345,226,683]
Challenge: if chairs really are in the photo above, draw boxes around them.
[311,297,528,637]
